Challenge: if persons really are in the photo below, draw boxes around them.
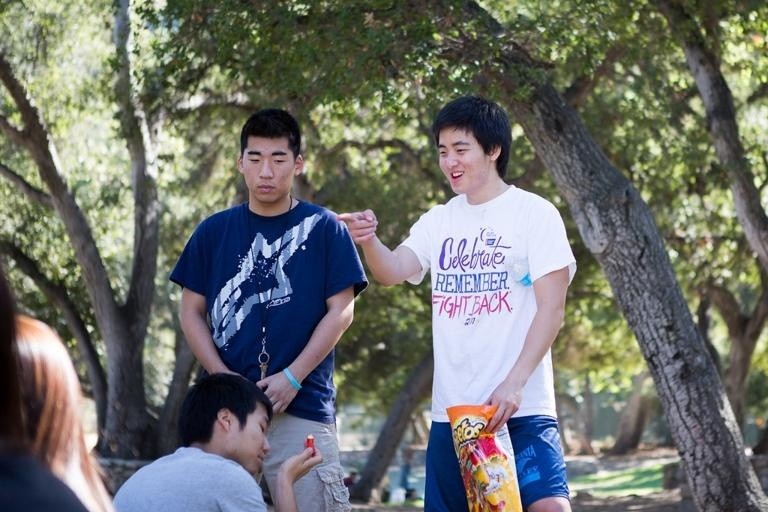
[11,313,118,512]
[112,373,322,512]
[166,110,370,512]
[335,97,576,511]
[0,260,92,512]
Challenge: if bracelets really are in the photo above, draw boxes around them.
[283,367,304,391]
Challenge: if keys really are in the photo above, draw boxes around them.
[258,363,270,382]
